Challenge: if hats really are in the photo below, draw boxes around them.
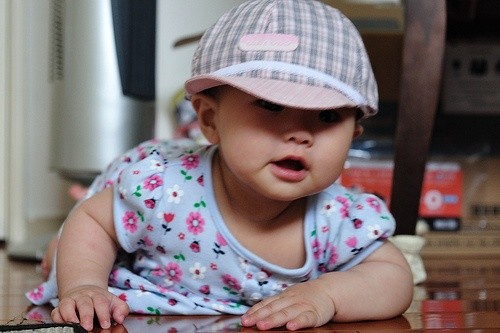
[185,0,379,119]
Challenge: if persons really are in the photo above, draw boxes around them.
[23,0,414,331]
